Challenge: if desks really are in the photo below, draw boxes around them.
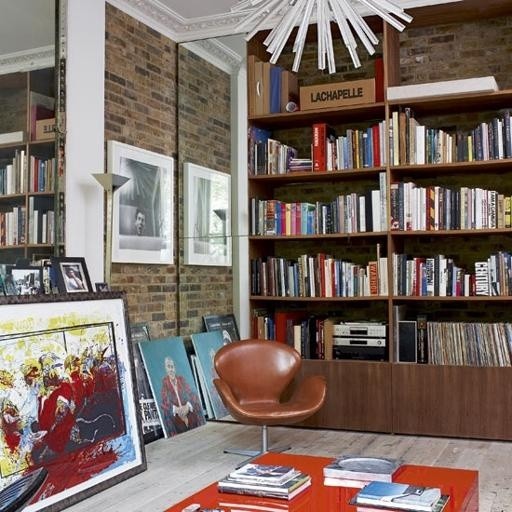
[163,449,480,512]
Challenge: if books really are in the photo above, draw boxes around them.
[249,243,389,297]
[249,309,335,361]
[1,91,65,247]
[249,120,386,175]
[393,305,512,368]
[249,171,388,236]
[389,180,511,231]
[393,250,512,296]
[389,108,510,166]
[181,455,451,512]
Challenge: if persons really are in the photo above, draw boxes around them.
[160,355,206,437]
[65,269,84,291]
[1,342,118,470]
[132,208,147,235]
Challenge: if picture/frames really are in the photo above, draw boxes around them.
[181,161,233,269]
[105,138,176,268]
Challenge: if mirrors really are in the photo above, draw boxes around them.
[174,31,244,424]
[0,1,69,295]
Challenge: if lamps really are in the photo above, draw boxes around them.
[229,0,414,75]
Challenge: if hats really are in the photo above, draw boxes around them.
[63,354,80,369]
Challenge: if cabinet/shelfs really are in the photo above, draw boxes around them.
[244,1,511,444]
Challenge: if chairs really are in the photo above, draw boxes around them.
[211,336,329,471]
